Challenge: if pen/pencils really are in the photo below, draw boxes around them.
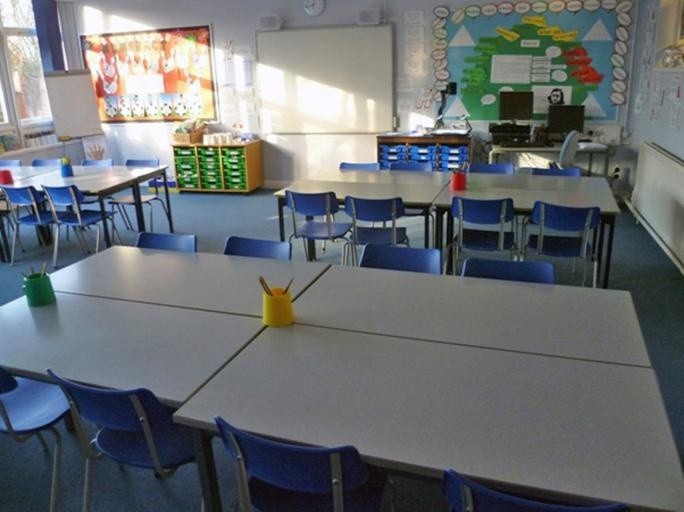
[31,267,35,275]
[259,275,272,295]
[283,277,294,297]
[20,271,28,278]
[41,261,48,277]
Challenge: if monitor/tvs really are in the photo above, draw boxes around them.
[548,105,585,134]
[499,91,534,130]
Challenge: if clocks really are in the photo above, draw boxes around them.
[303,0,325,17]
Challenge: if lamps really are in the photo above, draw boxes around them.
[438,81,457,116]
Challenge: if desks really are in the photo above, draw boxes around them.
[5,177,142,247]
[172,322,683,510]
[1,291,271,408]
[34,164,174,234]
[46,244,331,317]
[1,166,60,183]
[488,142,610,177]
[287,264,653,368]
[274,181,444,262]
[433,185,621,289]
[447,172,609,191]
[306,170,450,185]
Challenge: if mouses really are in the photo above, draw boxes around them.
[547,141,554,147]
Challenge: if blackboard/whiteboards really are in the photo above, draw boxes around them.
[255,24,397,136]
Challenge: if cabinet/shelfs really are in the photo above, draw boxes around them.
[171,139,263,193]
[377,135,473,172]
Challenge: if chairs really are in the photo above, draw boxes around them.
[40,184,118,269]
[0,365,71,512]
[223,235,292,261]
[213,415,383,512]
[460,257,556,284]
[358,243,442,274]
[0,159,22,167]
[46,367,206,512]
[285,190,353,266]
[343,194,410,267]
[467,161,515,176]
[339,162,381,228]
[134,231,197,253]
[107,158,170,242]
[530,167,579,177]
[450,196,519,262]
[78,159,130,243]
[389,163,435,249]
[1,185,69,267]
[439,468,626,512]
[32,159,61,166]
[521,200,601,289]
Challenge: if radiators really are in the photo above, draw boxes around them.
[629,138,684,276]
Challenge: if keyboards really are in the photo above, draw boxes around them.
[499,140,545,148]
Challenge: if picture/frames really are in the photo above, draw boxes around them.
[78,23,222,124]
